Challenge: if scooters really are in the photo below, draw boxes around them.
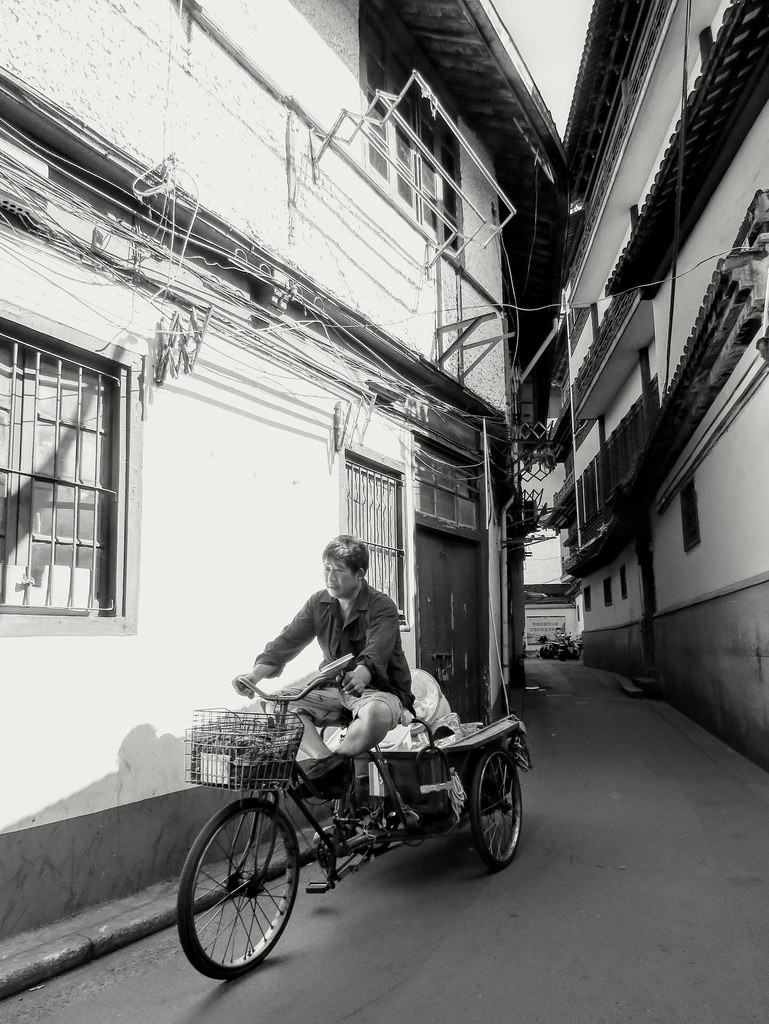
[537,627,582,662]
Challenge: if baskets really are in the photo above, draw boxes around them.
[182,708,305,791]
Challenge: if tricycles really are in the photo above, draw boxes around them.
[175,676,532,981]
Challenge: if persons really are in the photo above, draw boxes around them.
[231,535,416,801]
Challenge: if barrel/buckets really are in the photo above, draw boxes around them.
[409,668,451,735]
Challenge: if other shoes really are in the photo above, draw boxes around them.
[323,758,353,801]
[292,752,346,800]
